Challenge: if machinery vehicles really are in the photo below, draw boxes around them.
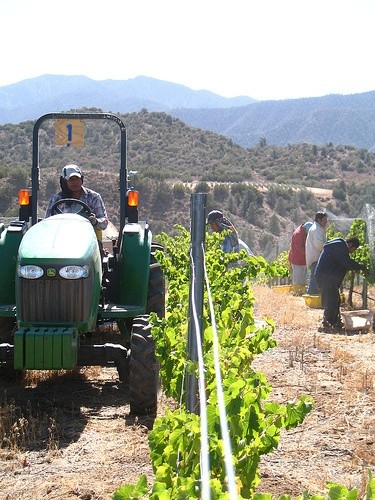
[1,112,170,418]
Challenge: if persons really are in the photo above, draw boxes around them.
[206,210,239,269]
[46,164,108,258]
[289,222,313,284]
[314,236,365,332]
[305,211,327,294]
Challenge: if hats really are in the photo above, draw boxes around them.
[61,164,81,180]
[206,210,223,225]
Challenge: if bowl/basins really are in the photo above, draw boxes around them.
[302,292,344,308]
